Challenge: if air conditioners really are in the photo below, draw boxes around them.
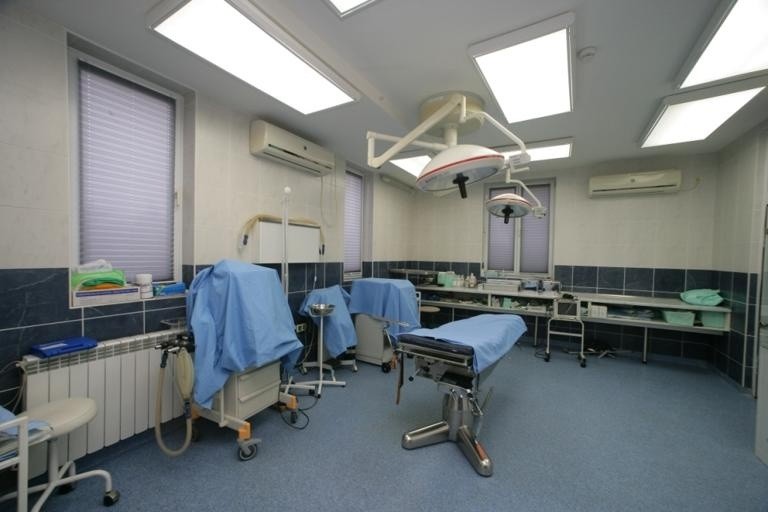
[584,165,681,199]
[249,116,338,179]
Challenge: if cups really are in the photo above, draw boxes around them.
[135,272,153,299]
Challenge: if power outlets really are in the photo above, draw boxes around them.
[694,176,702,185]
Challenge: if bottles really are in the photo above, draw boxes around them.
[452,272,477,288]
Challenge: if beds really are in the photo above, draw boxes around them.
[393,312,530,478]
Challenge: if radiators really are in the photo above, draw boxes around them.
[17,325,188,480]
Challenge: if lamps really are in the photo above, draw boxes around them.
[671,1,768,92]
[327,0,374,18]
[142,0,361,117]
[464,10,577,126]
[387,150,439,184]
[485,136,574,167]
[636,75,768,155]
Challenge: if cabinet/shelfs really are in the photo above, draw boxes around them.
[251,218,321,262]
[389,267,733,366]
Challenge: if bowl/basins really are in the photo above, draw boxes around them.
[307,303,336,315]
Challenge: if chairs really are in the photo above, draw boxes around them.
[0,353,122,511]
